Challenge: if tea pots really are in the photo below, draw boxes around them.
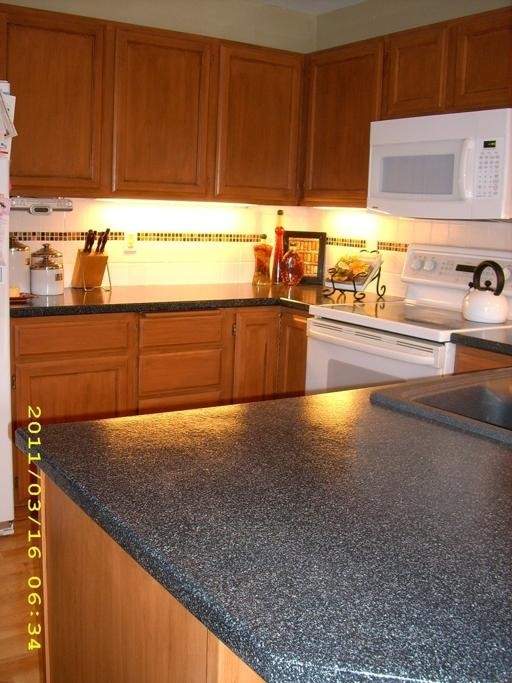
[462,260,507,323]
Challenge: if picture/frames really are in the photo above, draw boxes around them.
[284,231,325,284]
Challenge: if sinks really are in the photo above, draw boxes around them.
[370,365,512,445]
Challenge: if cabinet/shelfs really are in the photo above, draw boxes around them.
[384,6,512,117]
[11,313,130,520]
[130,307,282,414]
[107,20,304,206]
[300,33,385,207]
[283,307,310,394]
[455,344,512,373]
[0,3,107,198]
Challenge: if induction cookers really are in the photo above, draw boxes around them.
[309,301,512,333]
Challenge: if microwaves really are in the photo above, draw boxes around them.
[366,108,512,221]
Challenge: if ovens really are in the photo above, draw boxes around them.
[304,318,457,396]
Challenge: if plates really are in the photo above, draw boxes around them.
[9,293,34,301]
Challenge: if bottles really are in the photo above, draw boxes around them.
[281,242,304,285]
[271,210,285,286]
[252,234,271,284]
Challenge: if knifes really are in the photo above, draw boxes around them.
[83,229,111,254]
